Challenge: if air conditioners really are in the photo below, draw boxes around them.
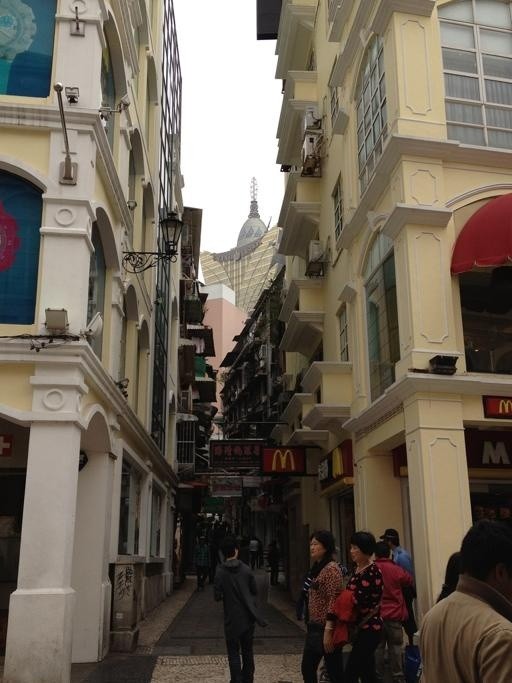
[305,235,331,277]
[259,360,265,370]
[234,390,238,400]
[301,109,327,144]
[298,135,328,178]
[258,344,267,359]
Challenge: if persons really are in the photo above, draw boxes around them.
[318,543,349,682]
[332,531,386,683]
[380,528,417,646]
[296,529,348,683]
[417,518,512,683]
[173,512,281,592]
[373,541,413,682]
[405,551,466,683]
[214,537,259,682]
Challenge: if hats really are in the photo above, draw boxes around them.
[379,529,398,539]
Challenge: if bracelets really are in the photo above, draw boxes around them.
[324,627,334,631]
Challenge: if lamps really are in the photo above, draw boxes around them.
[121,211,184,274]
